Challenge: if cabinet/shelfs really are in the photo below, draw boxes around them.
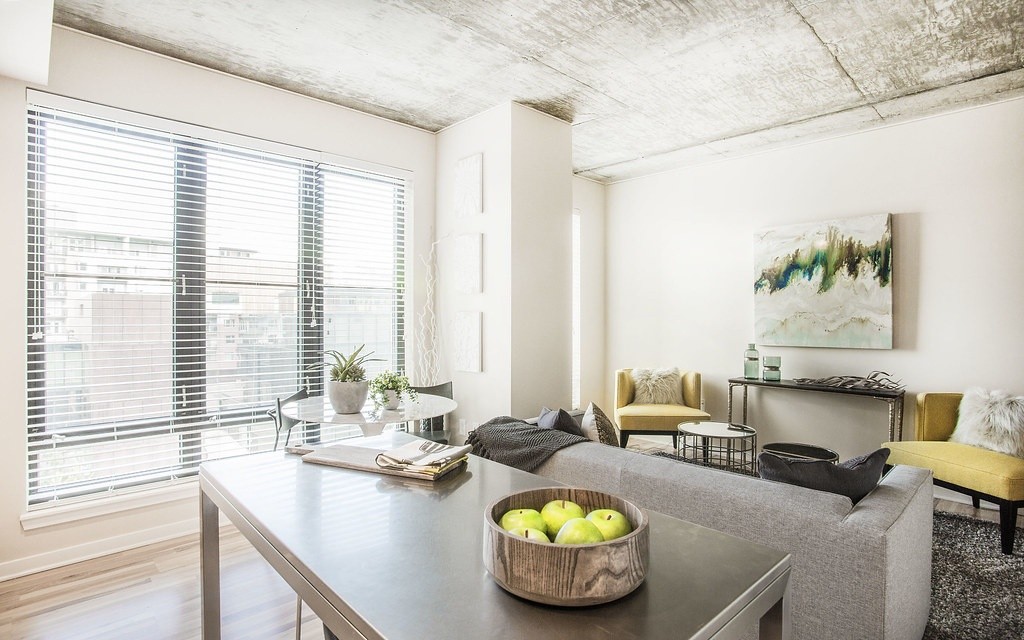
[727,375,905,441]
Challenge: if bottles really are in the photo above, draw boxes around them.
[743,343,759,380]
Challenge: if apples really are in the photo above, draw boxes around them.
[498,498,633,544]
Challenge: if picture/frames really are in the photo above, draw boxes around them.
[752,213,895,349]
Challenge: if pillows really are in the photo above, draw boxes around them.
[582,400,620,448]
[538,407,582,434]
[757,446,891,505]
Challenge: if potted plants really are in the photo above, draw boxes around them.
[306,343,387,415]
[367,369,420,419]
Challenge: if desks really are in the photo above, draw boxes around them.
[282,390,458,437]
[677,420,758,476]
[198,428,794,640]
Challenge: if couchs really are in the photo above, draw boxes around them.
[882,390,1024,554]
[614,366,712,461]
[465,408,933,640]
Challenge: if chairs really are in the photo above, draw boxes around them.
[409,381,454,445]
[266,385,309,450]
